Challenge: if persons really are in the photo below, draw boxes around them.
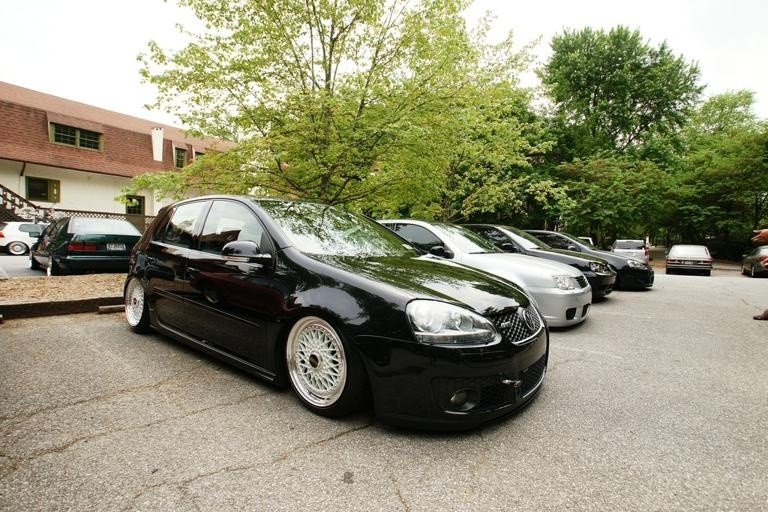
[750,228,768,321]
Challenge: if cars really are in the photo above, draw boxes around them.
[740,244,768,277]
[27,216,142,280]
[366,218,656,332]
[664,243,713,276]
[122,194,553,436]
[137,254,296,323]
[0,220,53,257]
